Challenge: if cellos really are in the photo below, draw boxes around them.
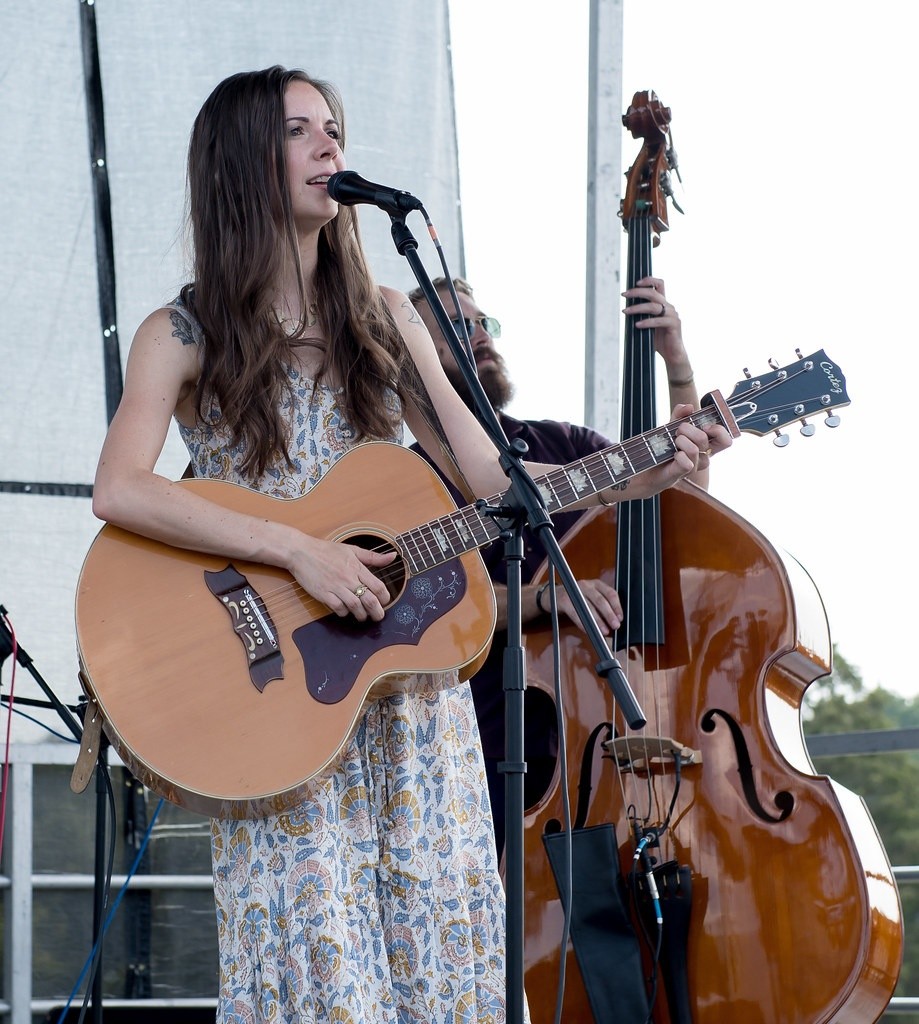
[493,132,908,1024]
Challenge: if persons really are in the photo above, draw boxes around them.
[407,276,709,872]
[92,64,732,1024]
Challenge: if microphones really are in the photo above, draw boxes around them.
[327,171,423,209]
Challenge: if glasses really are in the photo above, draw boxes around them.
[451,317,501,342]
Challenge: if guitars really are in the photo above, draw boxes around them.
[72,341,858,825]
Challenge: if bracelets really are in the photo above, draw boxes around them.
[598,492,616,507]
[536,580,558,616]
[668,371,695,388]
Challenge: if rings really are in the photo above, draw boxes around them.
[699,448,712,455]
[353,584,368,598]
[654,303,665,317]
